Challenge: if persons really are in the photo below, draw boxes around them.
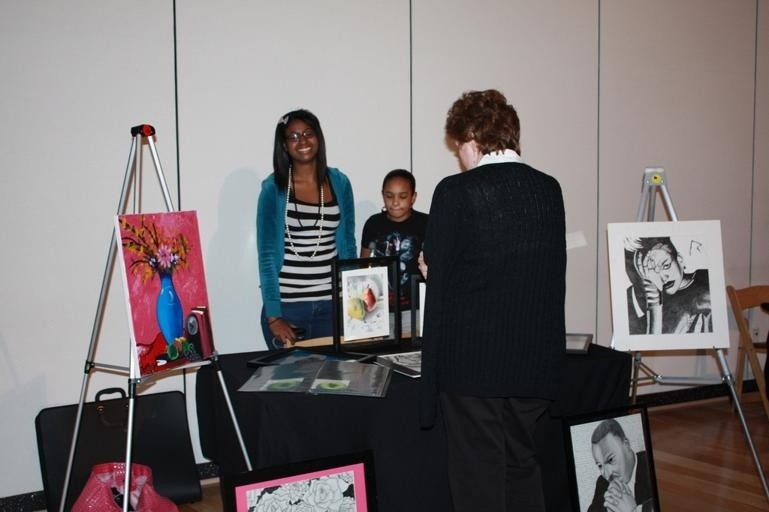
[359,168,429,339]
[588,421,657,512]
[256,108,356,350]
[418,88,570,511]
[625,237,712,335]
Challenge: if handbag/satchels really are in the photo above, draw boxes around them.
[71,461,178,512]
[35,388,203,511]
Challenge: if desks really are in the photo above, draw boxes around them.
[195,335,632,512]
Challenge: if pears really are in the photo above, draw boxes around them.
[346,296,366,326]
[363,285,377,312]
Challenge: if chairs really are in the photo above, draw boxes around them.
[726,285,769,417]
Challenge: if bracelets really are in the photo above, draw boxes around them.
[266,316,280,328]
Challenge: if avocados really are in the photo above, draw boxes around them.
[266,380,300,392]
[317,382,344,391]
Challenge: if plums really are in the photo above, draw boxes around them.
[155,353,168,361]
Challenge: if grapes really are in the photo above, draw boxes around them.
[182,341,202,362]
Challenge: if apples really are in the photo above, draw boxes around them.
[174,337,187,353]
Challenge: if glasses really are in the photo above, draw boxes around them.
[287,127,315,143]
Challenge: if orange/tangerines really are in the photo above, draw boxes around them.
[167,344,179,363]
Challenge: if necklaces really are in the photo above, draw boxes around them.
[284,162,326,262]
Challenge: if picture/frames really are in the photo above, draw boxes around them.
[223,450,378,512]
[411,272,427,350]
[331,255,402,349]
[562,402,660,512]
